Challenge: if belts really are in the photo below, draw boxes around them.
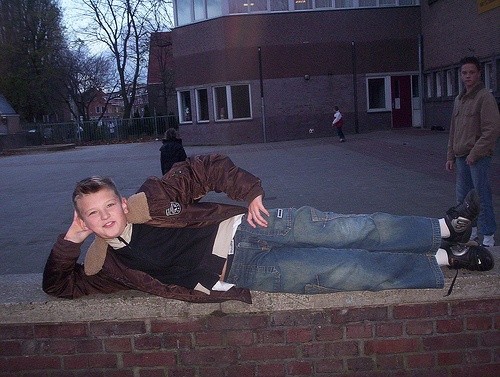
[222,239,235,283]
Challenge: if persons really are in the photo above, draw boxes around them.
[73,125,86,138]
[332,106,346,142]
[446,57,500,248]
[108,121,116,138]
[218,105,227,120]
[42,153,494,304]
[160,128,187,176]
[184,105,190,120]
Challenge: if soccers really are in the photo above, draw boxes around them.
[309,129,314,134]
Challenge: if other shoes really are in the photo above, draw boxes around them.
[480,233,495,248]
[339,138,346,143]
[469,227,479,241]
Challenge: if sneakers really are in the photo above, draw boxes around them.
[439,244,494,271]
[442,190,482,243]
